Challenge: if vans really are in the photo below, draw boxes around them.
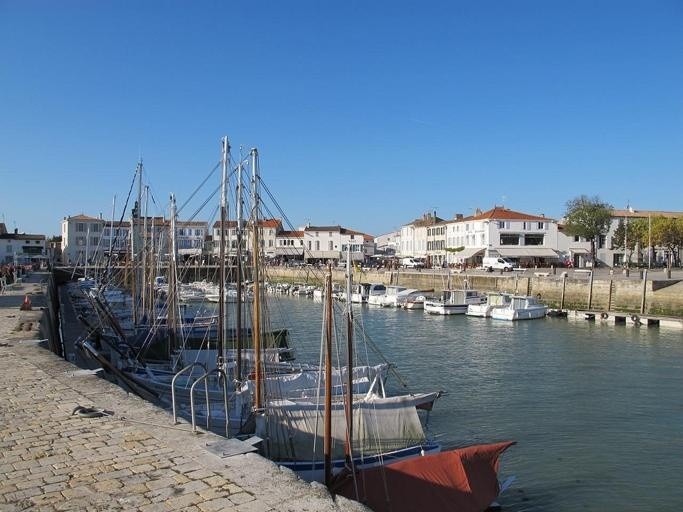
[483,257,513,272]
[403,258,424,269]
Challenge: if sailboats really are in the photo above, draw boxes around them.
[63,132,519,512]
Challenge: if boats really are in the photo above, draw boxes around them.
[491,277,548,321]
[423,279,487,315]
[464,279,517,318]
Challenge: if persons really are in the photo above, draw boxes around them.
[487,265,494,272]
[0,261,34,288]
[266,255,481,273]
